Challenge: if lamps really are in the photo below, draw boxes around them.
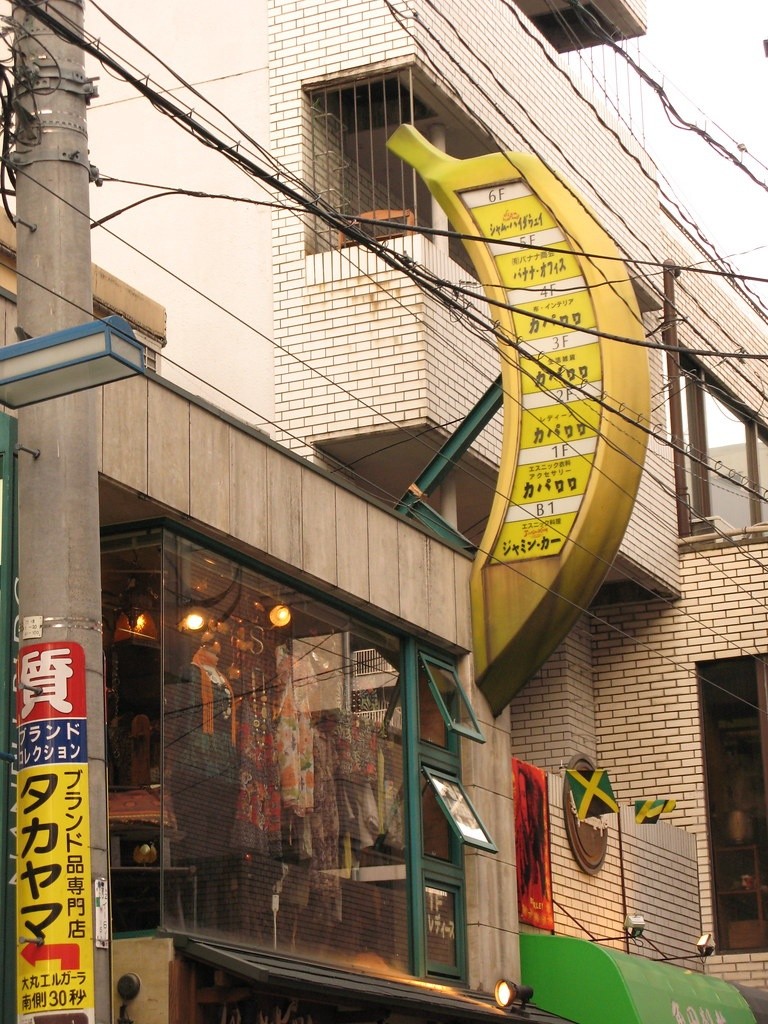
[652,934,716,962]
[495,979,535,1018]
[268,593,296,627]
[589,913,646,942]
[111,577,160,642]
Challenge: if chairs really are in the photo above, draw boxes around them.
[338,209,415,250]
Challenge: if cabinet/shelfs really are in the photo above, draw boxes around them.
[716,843,768,948]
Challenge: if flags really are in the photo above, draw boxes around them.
[562,770,676,824]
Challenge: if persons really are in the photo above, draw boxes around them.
[164,637,236,856]
